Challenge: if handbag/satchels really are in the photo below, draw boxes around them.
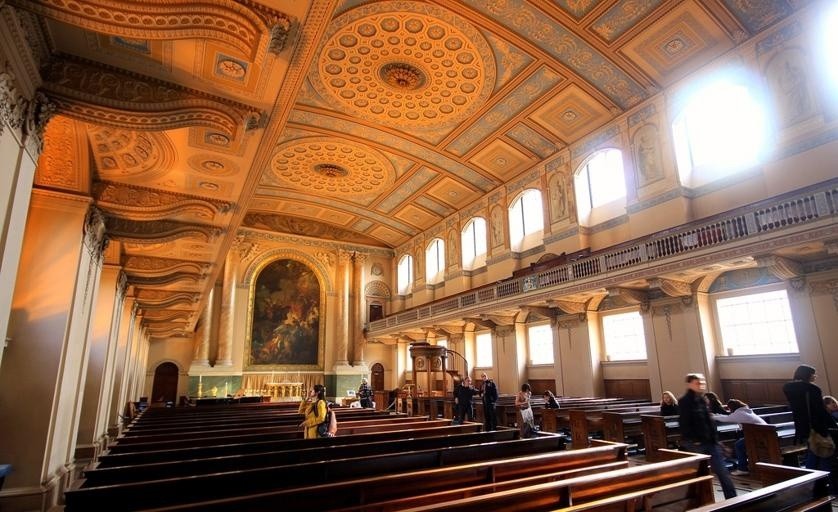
[808,428,836,458]
[521,406,534,423]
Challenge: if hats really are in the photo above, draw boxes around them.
[362,377,368,384]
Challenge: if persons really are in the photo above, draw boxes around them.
[781,367,838,494]
[453,376,483,423]
[702,392,729,415]
[710,398,768,475]
[358,380,375,408]
[479,374,498,431]
[297,385,327,440]
[822,395,838,426]
[518,422,539,439]
[515,383,534,431]
[678,374,736,499]
[543,390,560,408]
[661,391,680,415]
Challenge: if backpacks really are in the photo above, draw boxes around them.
[314,399,338,439]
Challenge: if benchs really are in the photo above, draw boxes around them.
[64,393,838,510]
